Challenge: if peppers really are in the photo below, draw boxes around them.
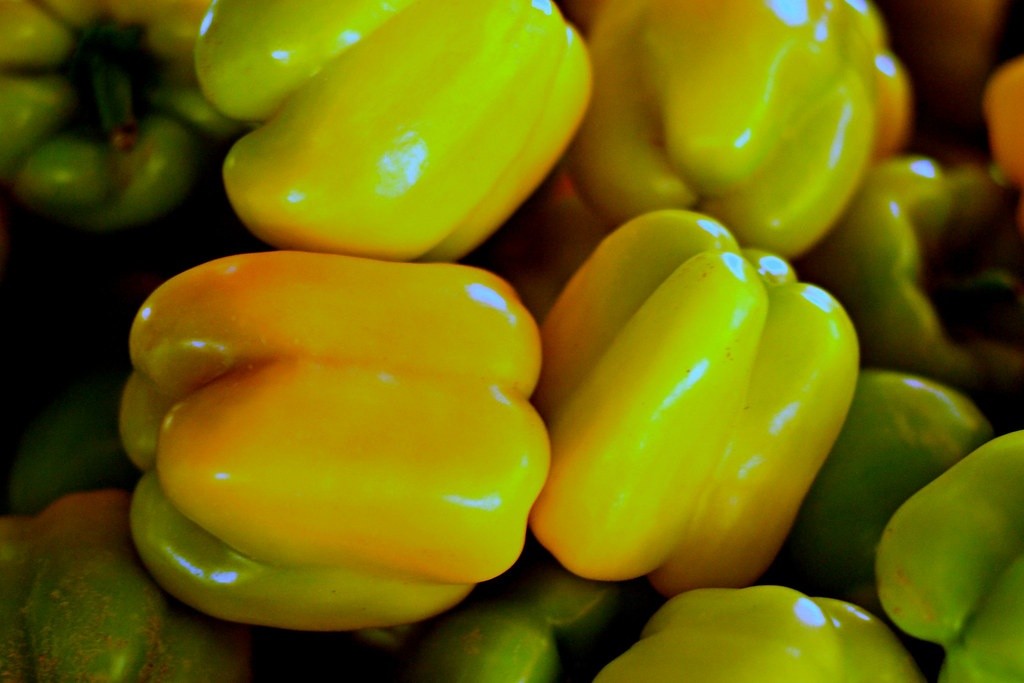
[0,0,1024,683]
